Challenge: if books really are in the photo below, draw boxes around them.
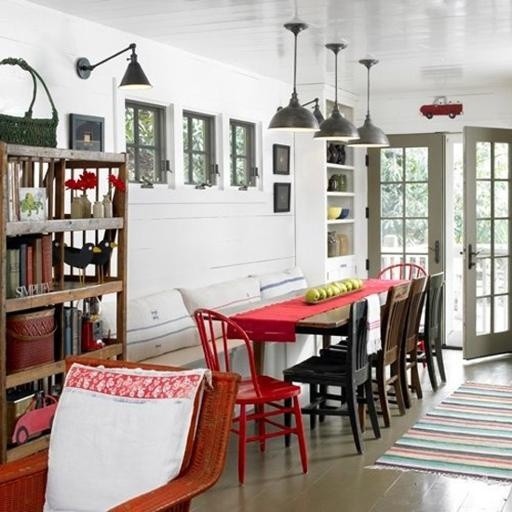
[9,163,20,222]
[7,236,54,299]
[64,307,82,357]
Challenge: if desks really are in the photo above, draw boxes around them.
[227,278,414,424]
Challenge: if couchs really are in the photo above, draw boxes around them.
[98,264,339,411]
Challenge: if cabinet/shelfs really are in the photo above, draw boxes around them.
[293,84,362,285]
[0,142,131,462]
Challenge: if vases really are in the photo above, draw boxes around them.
[80,194,90,218]
[102,194,113,218]
[92,200,105,219]
[70,195,83,217]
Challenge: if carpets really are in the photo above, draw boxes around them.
[360,381,512,485]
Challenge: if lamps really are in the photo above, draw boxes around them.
[265,21,322,136]
[75,40,155,94]
[314,40,361,145]
[346,56,394,150]
[276,96,325,126]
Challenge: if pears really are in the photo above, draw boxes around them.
[304,277,363,302]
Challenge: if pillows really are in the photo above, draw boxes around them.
[250,270,307,301]
[100,287,204,367]
[175,276,264,344]
[42,361,213,512]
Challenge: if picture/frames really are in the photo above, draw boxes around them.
[273,181,291,213]
[272,143,290,175]
[67,112,106,154]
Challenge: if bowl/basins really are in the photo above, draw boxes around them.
[326,206,350,219]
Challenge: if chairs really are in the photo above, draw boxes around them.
[318,261,447,432]
[282,292,381,456]
[192,307,309,486]
[0,352,244,512]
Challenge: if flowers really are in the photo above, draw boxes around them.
[102,172,126,195]
[76,170,97,193]
[65,176,82,196]
